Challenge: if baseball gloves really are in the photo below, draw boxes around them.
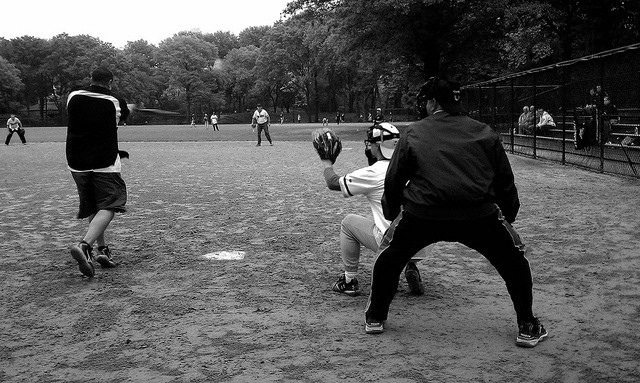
[311,128,343,165]
[19,128,25,134]
[252,124,256,128]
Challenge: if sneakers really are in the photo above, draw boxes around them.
[516,317,549,348]
[331,274,359,297]
[95,244,118,267]
[70,239,95,277]
[365,317,384,334]
[404,260,424,296]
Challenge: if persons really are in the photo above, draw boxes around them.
[279,110,286,125]
[322,116,329,125]
[65,66,128,277]
[210,112,219,131]
[367,112,373,123]
[374,108,386,124]
[390,110,396,122]
[586,84,621,117]
[365,80,548,346]
[204,112,210,129]
[5,115,26,145]
[336,111,342,124]
[252,104,272,147]
[189,112,197,127]
[297,112,302,124]
[313,124,433,295]
[519,104,556,135]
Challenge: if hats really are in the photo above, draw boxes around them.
[425,78,461,115]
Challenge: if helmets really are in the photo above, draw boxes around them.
[364,122,400,165]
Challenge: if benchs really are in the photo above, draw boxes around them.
[500,108,640,150]
